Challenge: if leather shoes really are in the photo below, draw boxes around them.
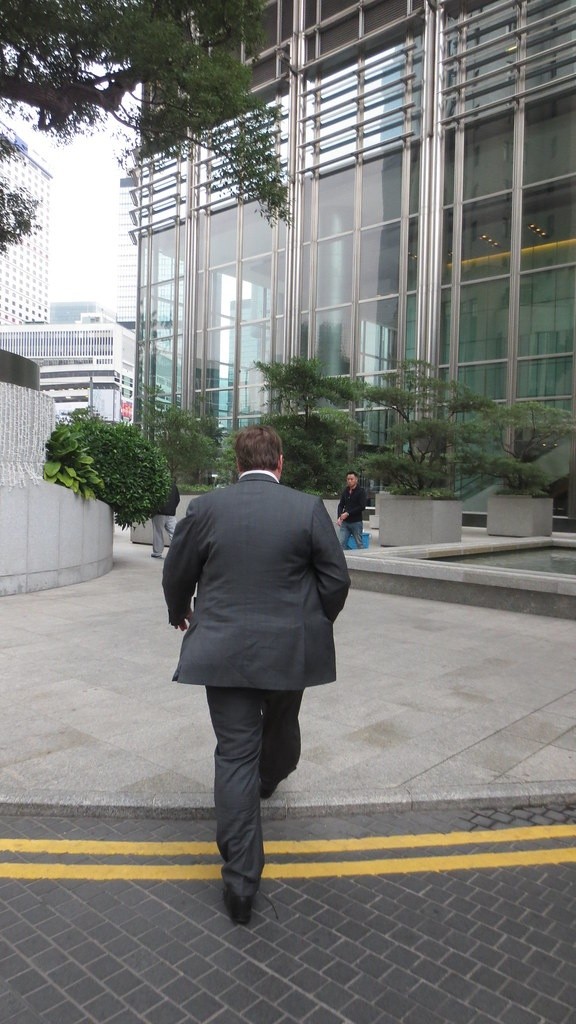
[224,888,256,924]
[258,773,279,798]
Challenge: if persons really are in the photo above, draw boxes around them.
[150,482,181,557]
[337,470,367,549]
[162,424,350,923]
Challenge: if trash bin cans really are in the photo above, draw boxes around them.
[347,532,370,550]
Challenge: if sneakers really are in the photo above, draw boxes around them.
[151,553,161,558]
